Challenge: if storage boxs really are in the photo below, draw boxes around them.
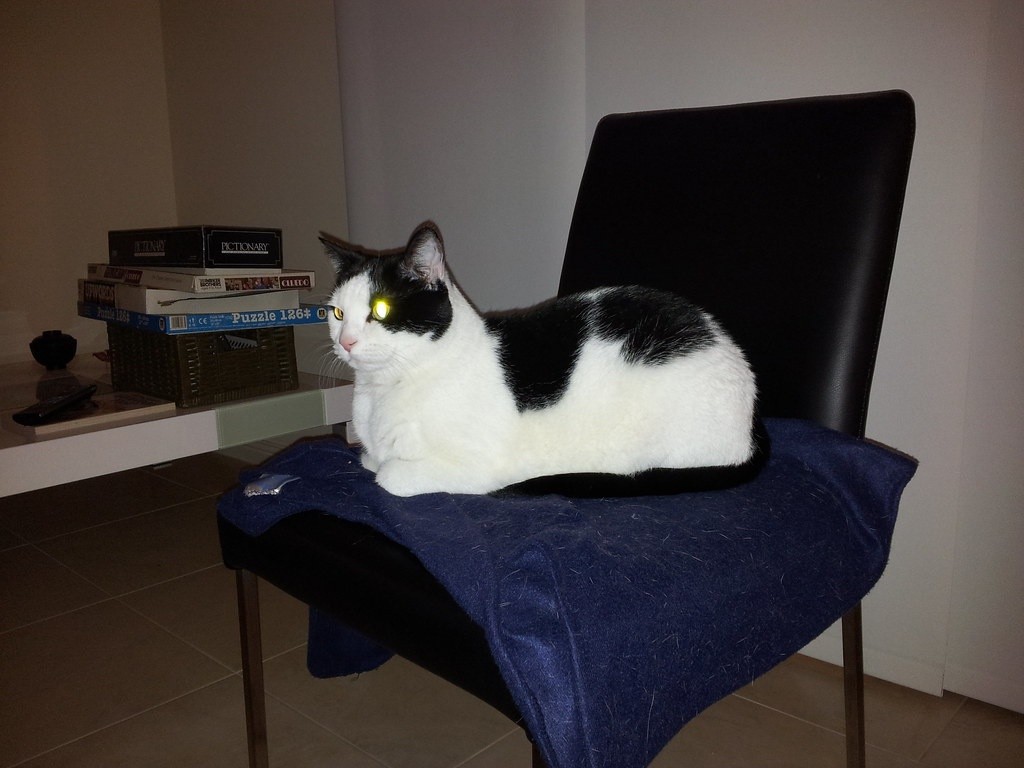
[77,225,327,409]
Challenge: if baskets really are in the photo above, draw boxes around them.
[106,321,298,409]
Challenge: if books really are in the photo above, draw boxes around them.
[77,263,328,336]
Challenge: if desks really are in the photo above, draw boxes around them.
[1,346,347,505]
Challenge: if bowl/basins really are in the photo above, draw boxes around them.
[28,328,78,372]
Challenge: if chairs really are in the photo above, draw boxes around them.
[216,86,922,768]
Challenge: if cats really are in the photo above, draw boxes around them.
[318,216,758,496]
